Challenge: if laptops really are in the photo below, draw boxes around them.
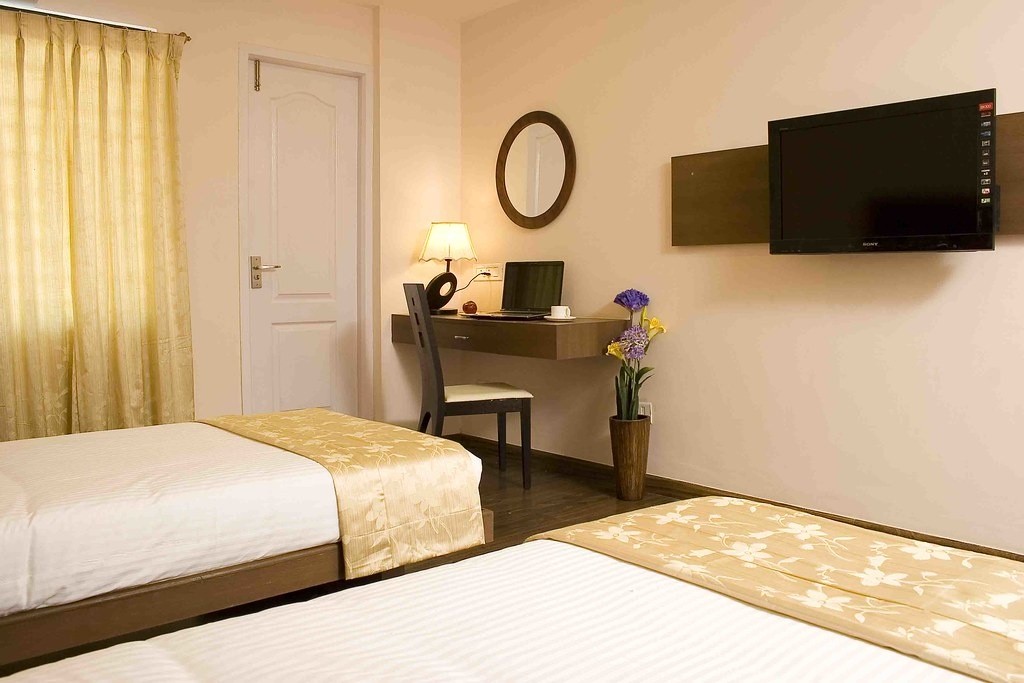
[469,261,565,321]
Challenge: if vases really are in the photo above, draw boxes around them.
[610,415,652,500]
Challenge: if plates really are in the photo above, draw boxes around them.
[544,316,576,321]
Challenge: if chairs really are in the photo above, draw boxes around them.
[404,283,534,489]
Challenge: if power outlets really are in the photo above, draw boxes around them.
[638,402,652,425]
[473,263,503,281]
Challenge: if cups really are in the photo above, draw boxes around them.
[551,306,570,317]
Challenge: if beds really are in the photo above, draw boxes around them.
[0,407,493,673]
[0,496,1024,683]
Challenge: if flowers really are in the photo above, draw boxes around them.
[606,289,666,420]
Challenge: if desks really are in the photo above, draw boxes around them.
[391,313,631,360]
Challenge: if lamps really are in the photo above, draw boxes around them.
[418,222,478,315]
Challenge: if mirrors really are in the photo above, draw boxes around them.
[495,111,577,230]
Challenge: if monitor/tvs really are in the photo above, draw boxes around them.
[768,87,996,255]
